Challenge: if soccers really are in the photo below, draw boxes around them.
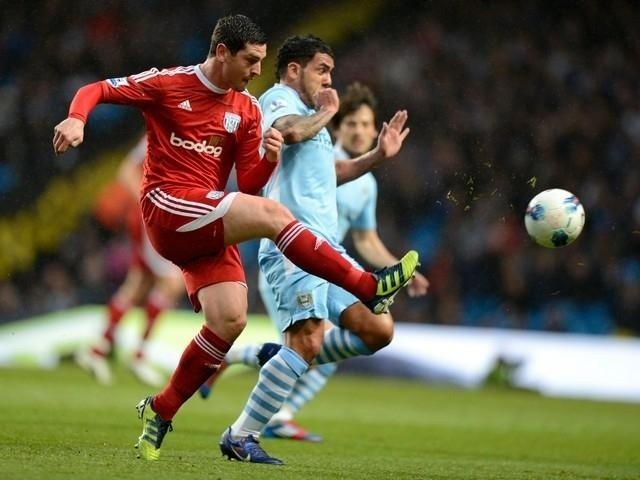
[525,188,585,247]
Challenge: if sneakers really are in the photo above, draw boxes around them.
[363,251,422,316]
[262,423,321,440]
[199,379,215,400]
[220,425,283,465]
[134,397,172,462]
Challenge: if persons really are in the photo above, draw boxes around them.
[75,132,188,390]
[52,15,418,464]
[201,32,410,465]
[265,82,429,441]
